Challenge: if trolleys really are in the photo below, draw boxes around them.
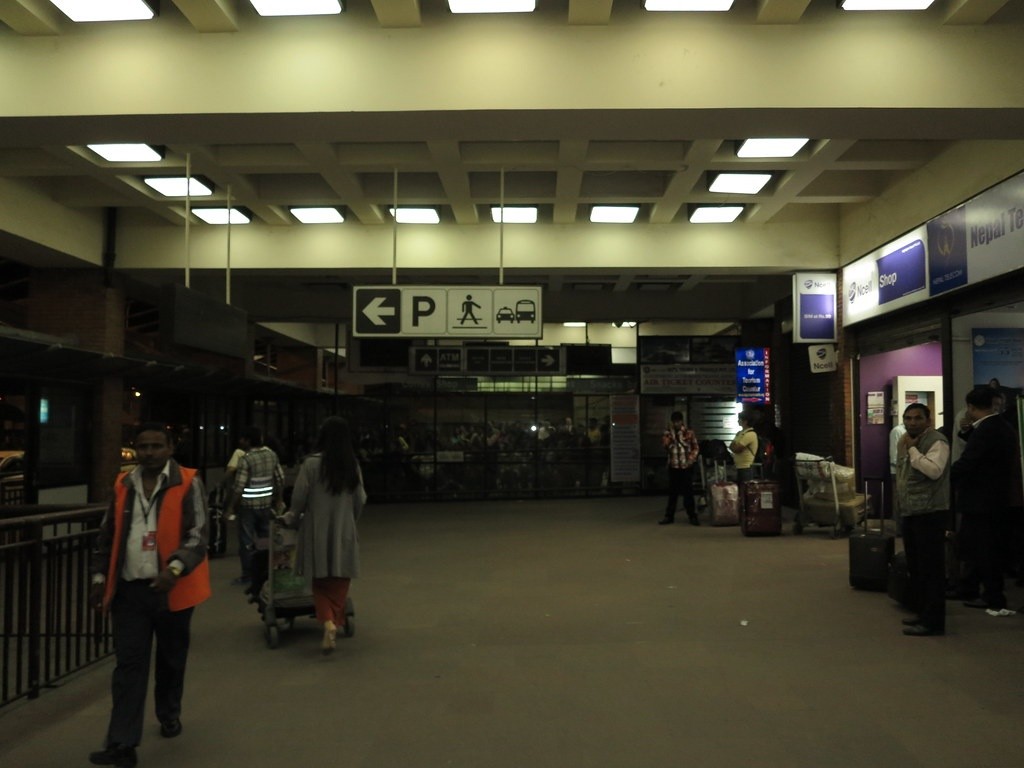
[247,513,358,647]
[791,455,867,540]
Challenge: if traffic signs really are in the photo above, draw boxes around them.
[352,282,545,342]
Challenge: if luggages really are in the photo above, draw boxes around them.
[708,457,741,527]
[207,481,228,558]
[739,463,783,537]
[848,477,896,592]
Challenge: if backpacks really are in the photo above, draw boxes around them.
[743,429,768,463]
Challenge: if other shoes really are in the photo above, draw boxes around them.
[322,625,338,653]
[963,592,1008,610]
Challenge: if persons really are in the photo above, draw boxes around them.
[215,427,282,583]
[282,415,367,652]
[755,431,785,473]
[730,411,758,500]
[894,387,1022,636]
[399,416,609,489]
[89,420,208,766]
[658,411,699,525]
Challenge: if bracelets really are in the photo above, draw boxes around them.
[171,568,179,576]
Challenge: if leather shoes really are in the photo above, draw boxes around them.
[88,743,138,768]
[689,518,700,526]
[902,617,930,625]
[902,623,945,636]
[160,718,182,738]
[658,516,674,525]
